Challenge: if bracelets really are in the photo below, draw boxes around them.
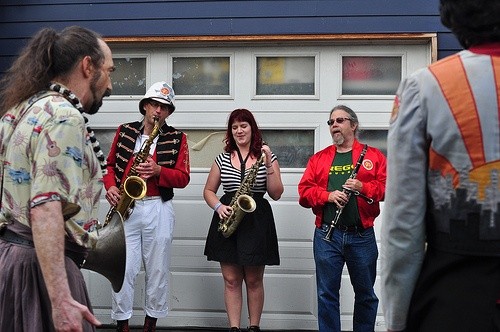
[214,202,222,210]
[266,165,272,168]
[267,172,274,175]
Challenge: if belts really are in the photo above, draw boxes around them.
[0,228,32,247]
[335,224,364,232]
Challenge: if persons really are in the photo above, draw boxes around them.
[203,109,284,332]
[103,82,190,332]
[0,27,116,332]
[298,105,386,332]
[381,0,500,332]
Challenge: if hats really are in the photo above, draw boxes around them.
[139,82,176,115]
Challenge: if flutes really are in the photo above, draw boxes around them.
[321,145,367,242]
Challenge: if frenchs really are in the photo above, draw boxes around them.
[0,158,127,293]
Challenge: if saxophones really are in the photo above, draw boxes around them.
[105,115,160,229]
[217,141,268,239]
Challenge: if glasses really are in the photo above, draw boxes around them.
[327,118,350,125]
[149,102,168,110]
